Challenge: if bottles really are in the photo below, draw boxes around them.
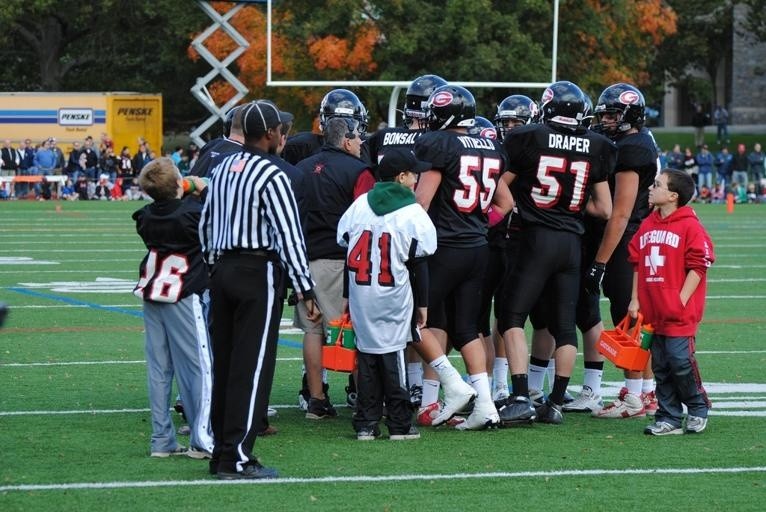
[325,318,356,350]
[182,175,210,194]
[727,192,734,213]
[639,323,654,351]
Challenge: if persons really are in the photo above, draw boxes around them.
[658,140,766,205]
[197,95,322,480]
[175,73,665,439]
[714,103,733,145]
[129,154,216,461]
[0,135,205,204]
[627,168,716,439]
[689,104,712,147]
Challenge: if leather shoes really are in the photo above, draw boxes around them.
[217,464,279,479]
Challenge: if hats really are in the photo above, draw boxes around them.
[240,98,295,131]
[378,147,433,176]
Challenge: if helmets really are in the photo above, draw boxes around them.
[222,106,241,137]
[402,76,647,131]
[321,89,367,129]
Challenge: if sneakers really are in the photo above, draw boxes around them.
[389,427,420,441]
[591,389,643,420]
[175,399,191,436]
[298,376,328,407]
[150,443,188,459]
[188,445,212,459]
[644,419,685,436]
[346,384,574,434]
[641,391,654,414]
[305,396,335,418]
[683,412,708,433]
[560,387,603,411]
[355,430,381,440]
